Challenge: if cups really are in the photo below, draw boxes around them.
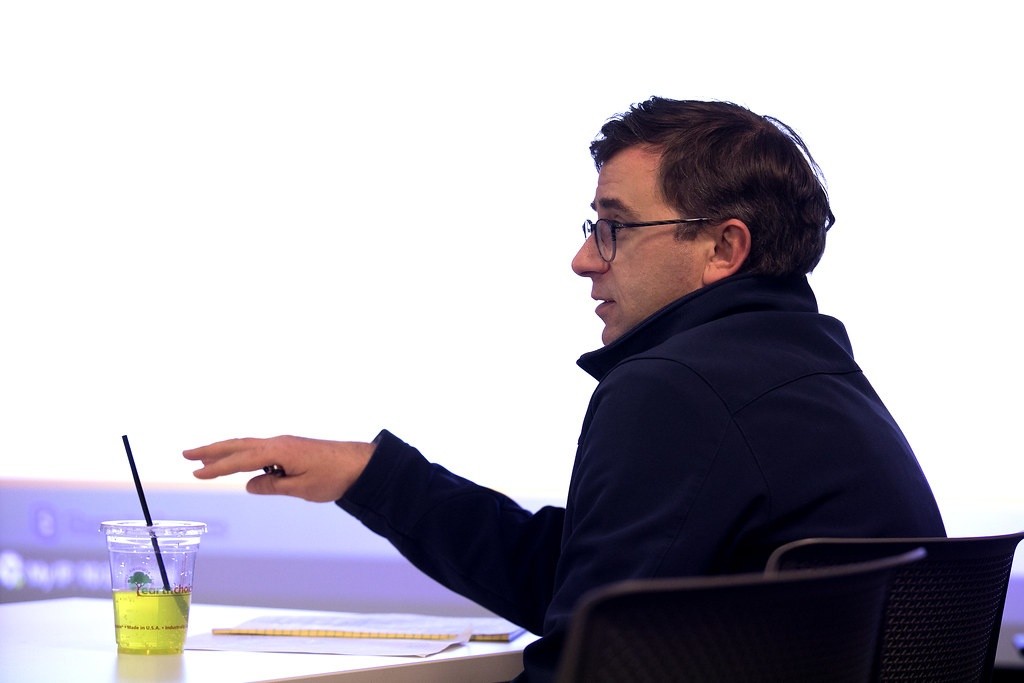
[98,519,208,654]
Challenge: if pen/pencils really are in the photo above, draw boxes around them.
[262,463,285,478]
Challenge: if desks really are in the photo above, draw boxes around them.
[2,598,545,683]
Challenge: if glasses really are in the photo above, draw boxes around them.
[582,218,708,262]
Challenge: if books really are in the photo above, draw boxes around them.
[211,613,527,643]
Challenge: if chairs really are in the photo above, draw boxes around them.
[556,531,1023,683]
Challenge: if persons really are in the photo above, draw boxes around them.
[182,97,948,683]
